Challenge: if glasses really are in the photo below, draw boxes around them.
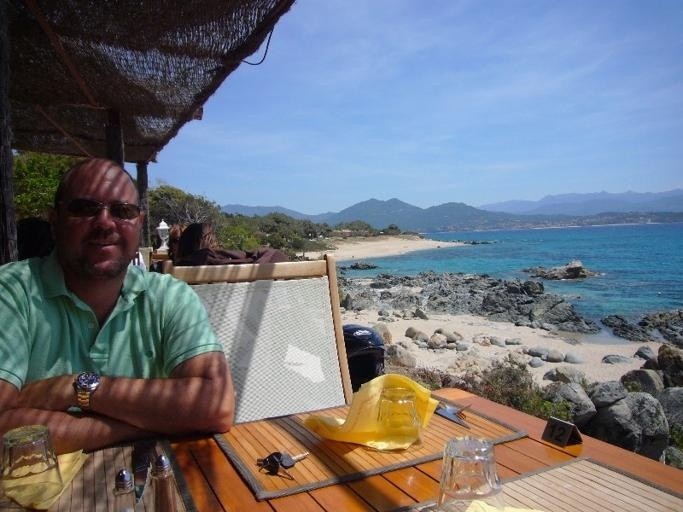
[66,198,141,220]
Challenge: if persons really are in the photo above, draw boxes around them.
[163,213,285,263]
[0,157,235,461]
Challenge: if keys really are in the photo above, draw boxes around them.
[257,450,312,478]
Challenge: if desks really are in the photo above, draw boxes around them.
[0,388,683,512]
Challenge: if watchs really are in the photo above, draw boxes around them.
[72,372,100,408]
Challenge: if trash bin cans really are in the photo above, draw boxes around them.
[342,325,385,393]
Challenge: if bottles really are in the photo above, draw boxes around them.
[112,454,180,512]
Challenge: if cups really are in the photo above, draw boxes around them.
[436,436,503,512]
[373,388,419,442]
[0,424,65,506]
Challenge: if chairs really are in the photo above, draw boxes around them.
[154,256,351,426]
